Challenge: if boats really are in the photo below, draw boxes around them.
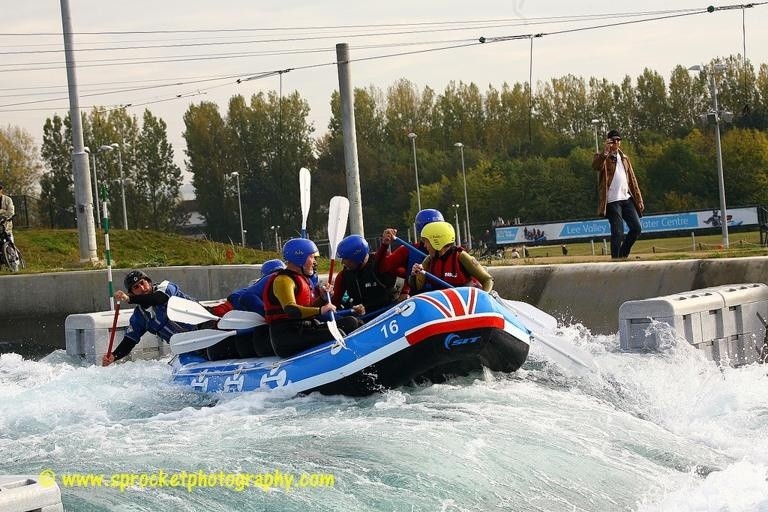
[166,280,540,407]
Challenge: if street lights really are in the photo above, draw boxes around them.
[66,141,133,234]
[229,172,248,249]
[451,203,463,251]
[453,141,475,254]
[407,131,422,216]
[270,225,282,251]
[685,61,736,252]
[588,116,603,216]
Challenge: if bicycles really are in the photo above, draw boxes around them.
[0,216,29,274]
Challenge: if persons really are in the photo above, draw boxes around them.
[0,184,20,267]
[212,258,288,318]
[263,238,367,361]
[590,128,645,259]
[511,247,520,259]
[373,206,446,302]
[100,270,235,365]
[408,220,495,296]
[559,240,567,256]
[330,233,411,324]
[311,253,322,295]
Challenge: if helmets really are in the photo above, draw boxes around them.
[335,233,371,263]
[413,207,446,234]
[123,269,152,291]
[419,219,456,251]
[282,237,321,268]
[260,258,287,276]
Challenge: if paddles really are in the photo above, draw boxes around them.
[165,295,267,355]
[416,265,594,374]
[324,283,346,350]
[391,234,557,336]
[299,167,311,239]
[325,196,351,283]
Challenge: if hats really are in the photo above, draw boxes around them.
[607,130,621,138]
